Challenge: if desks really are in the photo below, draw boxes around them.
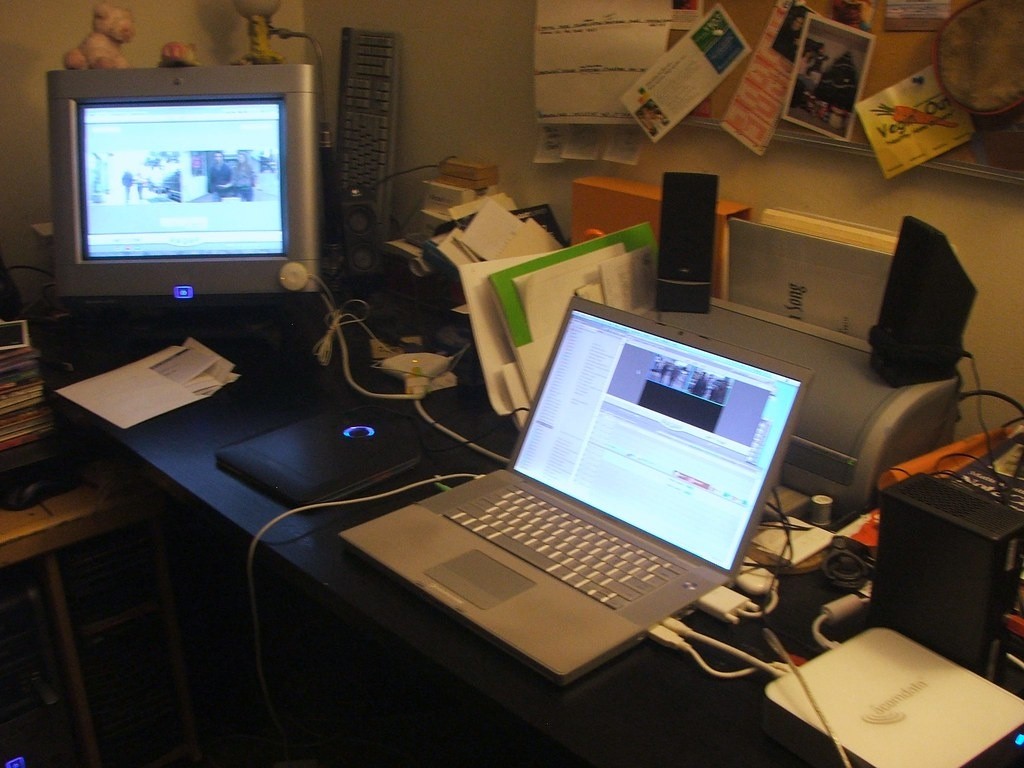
[45,335,1024,768]
[0,457,203,768]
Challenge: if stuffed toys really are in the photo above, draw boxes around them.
[62,3,136,69]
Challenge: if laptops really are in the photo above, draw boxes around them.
[215,403,423,508]
[335,294,816,688]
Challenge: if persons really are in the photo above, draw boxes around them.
[120,151,257,203]
[659,363,728,403]
[635,12,862,138]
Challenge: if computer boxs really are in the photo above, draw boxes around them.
[866,470,1024,686]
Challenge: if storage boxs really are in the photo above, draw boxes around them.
[421,179,499,216]
[418,209,449,237]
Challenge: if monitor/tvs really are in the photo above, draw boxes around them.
[47,61,345,315]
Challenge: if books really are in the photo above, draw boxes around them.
[0,344,56,452]
[487,222,658,433]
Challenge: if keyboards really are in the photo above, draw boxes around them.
[333,25,400,245]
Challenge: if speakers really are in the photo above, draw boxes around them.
[870,215,979,390]
[338,202,383,277]
[655,170,719,313]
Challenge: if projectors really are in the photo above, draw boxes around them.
[763,629,1024,768]
